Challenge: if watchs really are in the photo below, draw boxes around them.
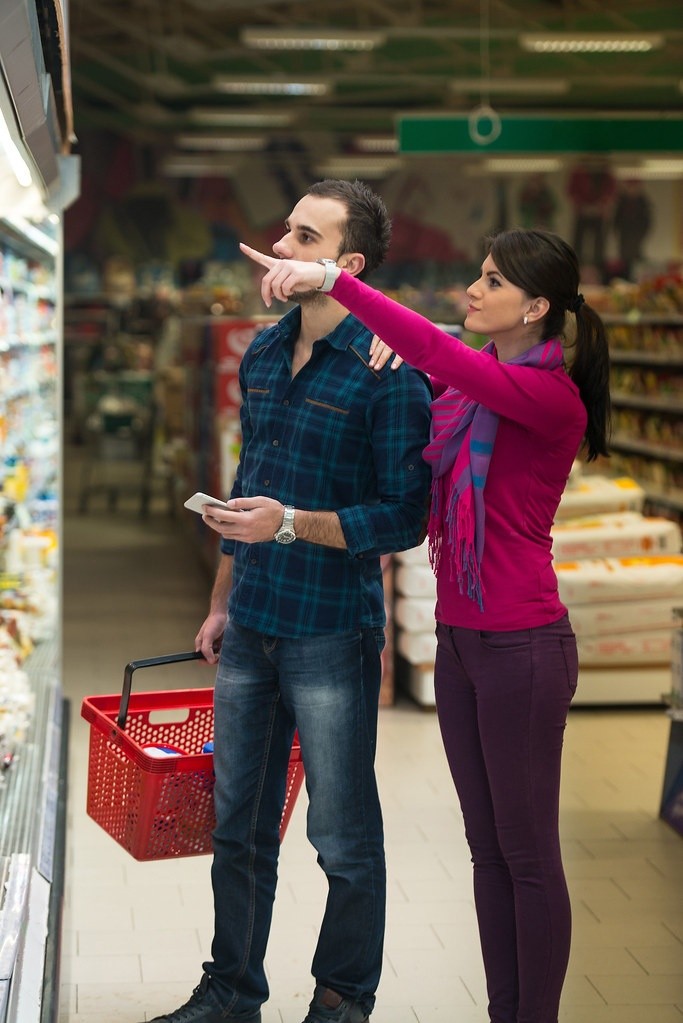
[315,258,337,292]
[273,504,296,545]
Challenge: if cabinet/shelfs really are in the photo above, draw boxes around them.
[73,313,683,709]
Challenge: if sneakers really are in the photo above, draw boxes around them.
[148,974,261,1023]
[302,984,371,1023]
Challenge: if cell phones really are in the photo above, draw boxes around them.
[184,491,244,522]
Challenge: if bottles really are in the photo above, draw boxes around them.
[186,743,216,836]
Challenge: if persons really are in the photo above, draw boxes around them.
[238,228,613,1023]
[138,178,433,1023]
[518,170,651,281]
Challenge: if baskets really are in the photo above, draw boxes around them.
[81,686,306,863]
